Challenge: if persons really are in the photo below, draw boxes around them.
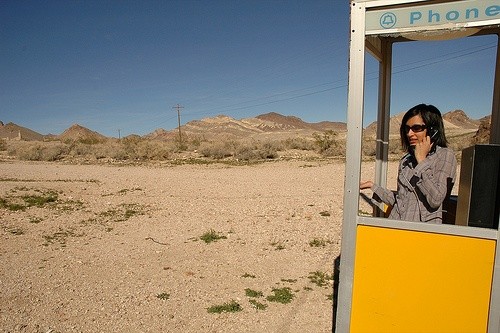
[358,104,457,227]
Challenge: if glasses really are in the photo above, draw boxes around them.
[404,124,427,133]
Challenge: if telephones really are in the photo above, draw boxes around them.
[408,128,440,155]
